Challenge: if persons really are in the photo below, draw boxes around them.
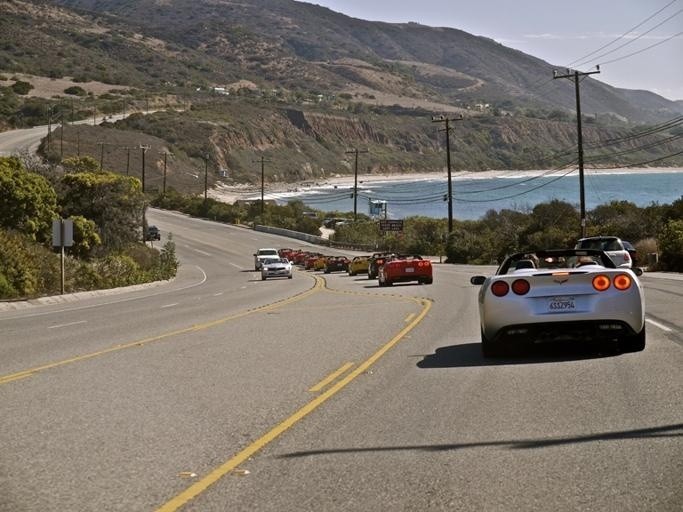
[522,253,539,269]
[573,255,591,269]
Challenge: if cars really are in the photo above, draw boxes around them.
[348,253,433,286]
[575,236,637,269]
[253,248,280,271]
[261,257,294,280]
[278,249,351,273]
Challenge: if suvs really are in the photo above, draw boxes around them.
[148,226,160,240]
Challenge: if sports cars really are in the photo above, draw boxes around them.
[471,250,645,351]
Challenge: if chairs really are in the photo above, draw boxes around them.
[515,258,534,269]
